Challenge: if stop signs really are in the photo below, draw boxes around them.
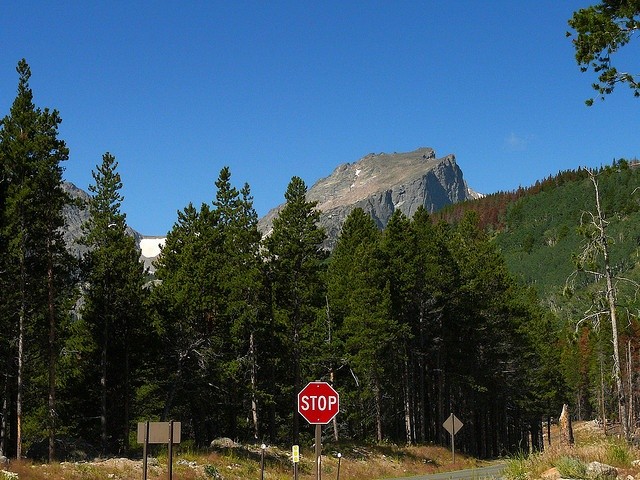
[297,382,339,424]
